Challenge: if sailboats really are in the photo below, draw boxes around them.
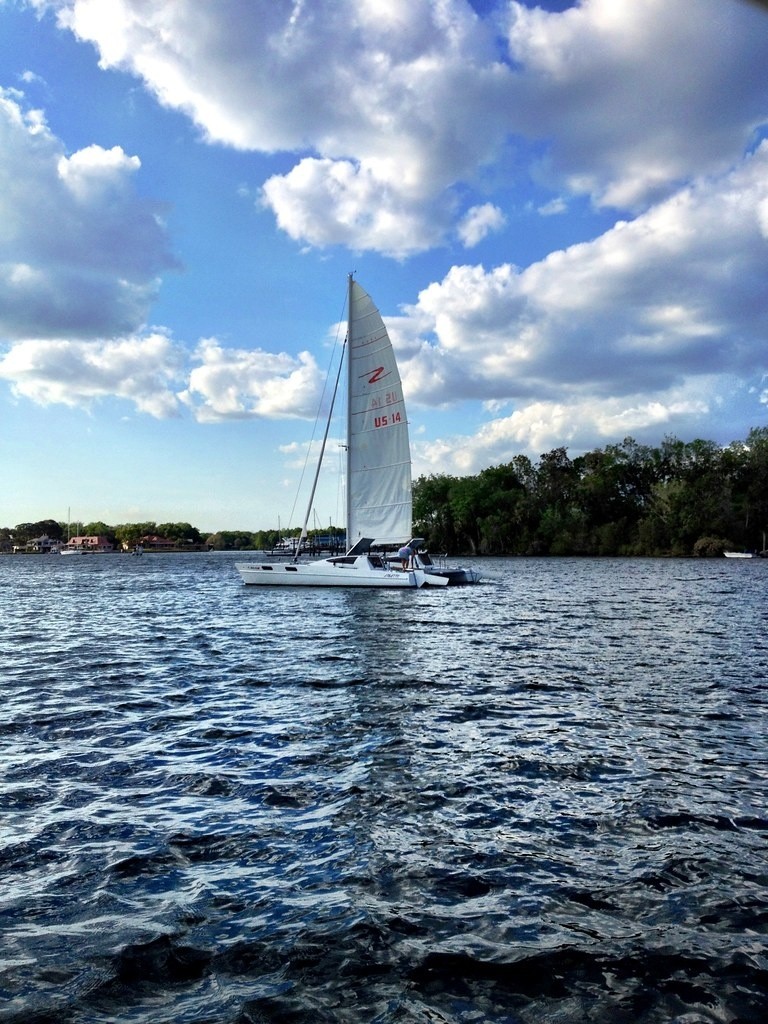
[233,270,482,588]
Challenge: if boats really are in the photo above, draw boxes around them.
[61,551,82,556]
[723,551,756,559]
[263,515,311,557]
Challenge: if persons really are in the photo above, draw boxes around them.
[398,545,415,570]
